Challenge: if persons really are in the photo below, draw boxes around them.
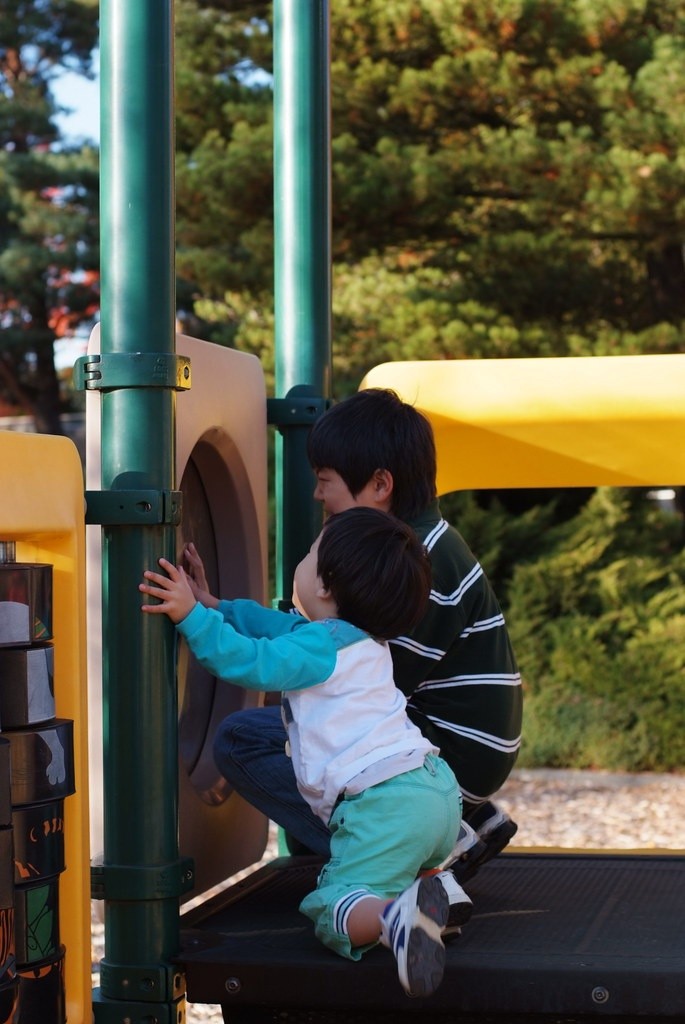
[210,387,524,885]
[138,506,473,997]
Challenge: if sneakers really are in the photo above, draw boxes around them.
[379,878,449,999]
[444,818,486,874]
[460,801,518,885]
[435,869,474,943]
